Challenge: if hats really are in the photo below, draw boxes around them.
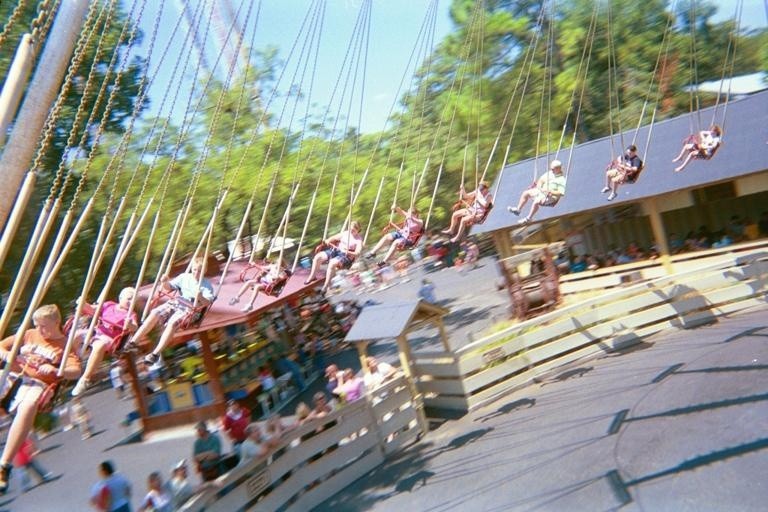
[550,160,561,169]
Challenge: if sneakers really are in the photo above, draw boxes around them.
[377,261,386,266]
[228,297,238,304]
[123,341,137,352]
[242,304,253,312]
[451,237,460,242]
[364,251,376,259]
[441,230,454,235]
[608,193,617,200]
[509,206,519,215]
[304,277,316,285]
[519,218,530,226]
[0,463,13,492]
[72,378,93,397]
[601,187,610,193]
[320,284,328,293]
[145,353,160,365]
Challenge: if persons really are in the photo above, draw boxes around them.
[136,354,168,391]
[543,215,768,275]
[71,287,138,395]
[296,357,398,486]
[90,462,132,512]
[172,294,366,417]
[119,257,214,366]
[366,206,423,266]
[0,304,81,489]
[194,400,298,510]
[111,363,124,400]
[141,459,219,512]
[506,159,568,225]
[13,438,54,492]
[600,145,644,200]
[304,221,362,295]
[70,397,92,440]
[673,121,723,174]
[442,186,492,242]
[229,259,287,313]
[419,279,436,304]
[328,232,479,293]
